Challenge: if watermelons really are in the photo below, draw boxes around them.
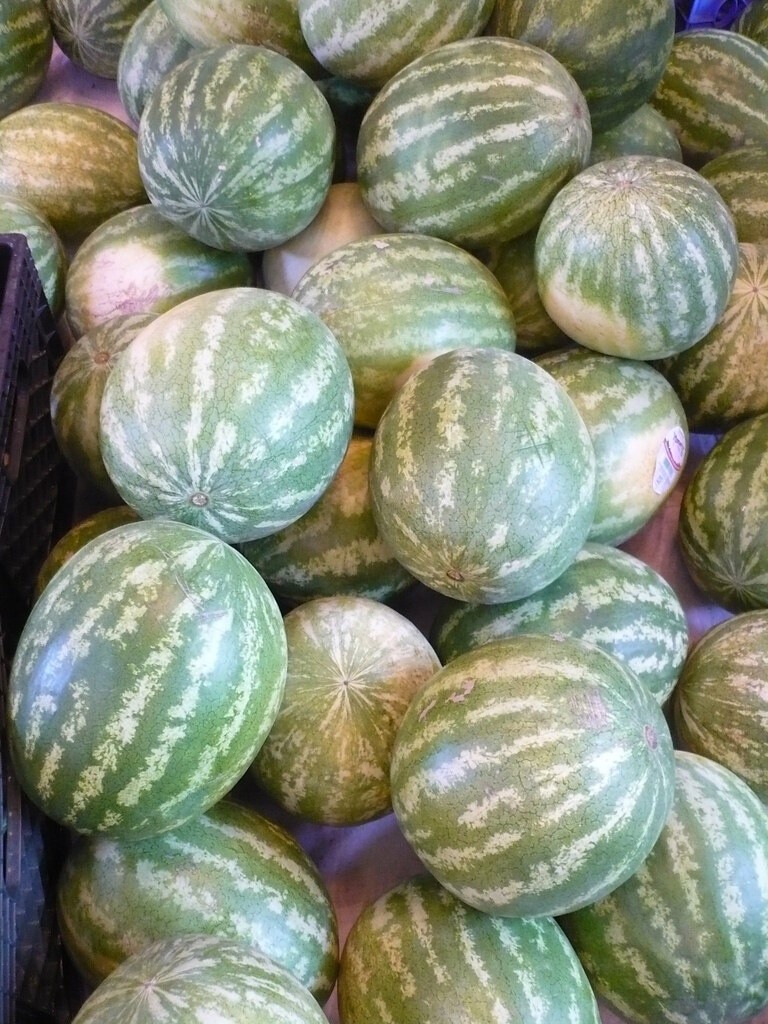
[0,0,768,1024]
